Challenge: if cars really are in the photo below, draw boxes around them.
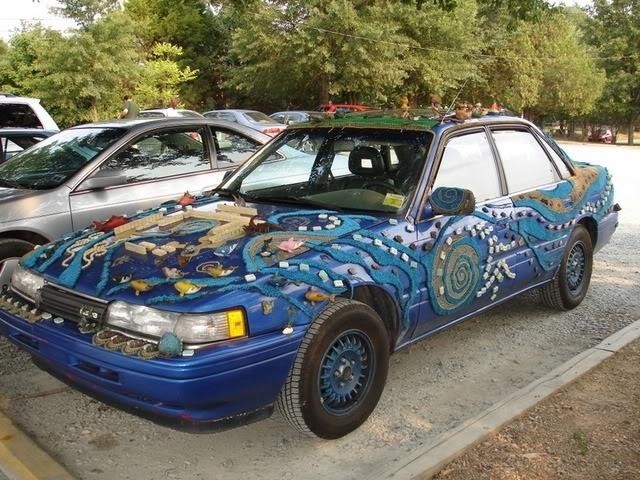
[2,103,625,443]
[588,128,614,145]
[1,117,360,299]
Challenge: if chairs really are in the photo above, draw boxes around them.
[317,133,544,231]
[22,137,175,190]
